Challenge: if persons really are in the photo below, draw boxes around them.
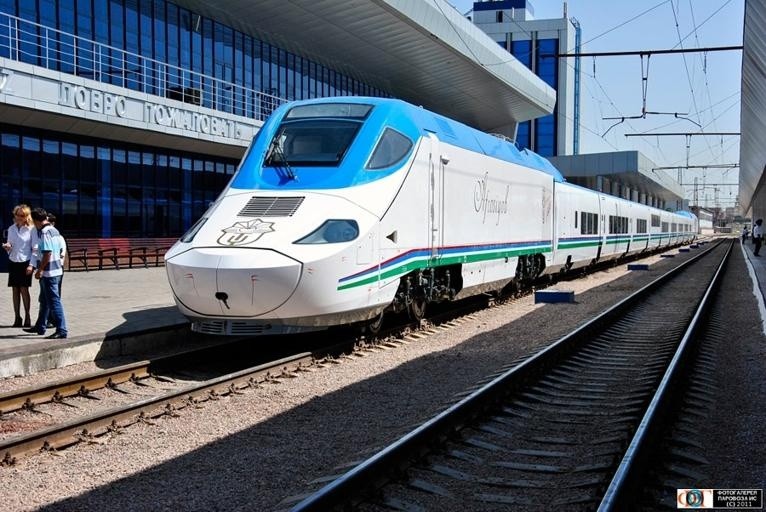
[753,219,764,256]
[23,207,68,339]
[2,204,39,328]
[46,213,67,329]
[751,224,756,244]
[742,226,750,244]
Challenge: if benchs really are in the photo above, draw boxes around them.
[66,237,181,272]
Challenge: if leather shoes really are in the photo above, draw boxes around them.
[47,322,54,328]
[23,327,44,335]
[46,332,66,339]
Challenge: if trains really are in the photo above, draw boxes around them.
[165,96,701,342]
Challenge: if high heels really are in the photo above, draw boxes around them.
[13,317,30,327]
